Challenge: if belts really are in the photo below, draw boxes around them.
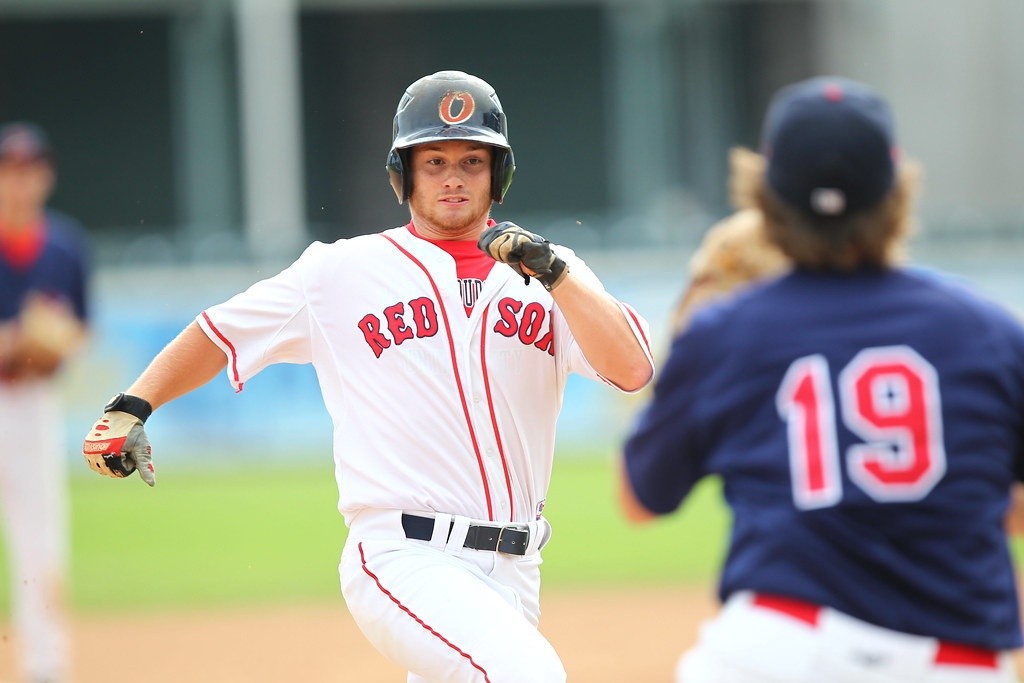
[752,590,998,669]
[402,513,530,558]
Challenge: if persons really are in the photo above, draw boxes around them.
[83,70,660,683]
[615,74,1024,683]
[0,121,95,683]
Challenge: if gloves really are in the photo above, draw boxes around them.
[84,392,155,487]
[476,220,571,293]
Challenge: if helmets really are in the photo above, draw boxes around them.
[0,118,46,164]
[386,69,516,203]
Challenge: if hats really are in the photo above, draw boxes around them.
[761,77,897,233]
